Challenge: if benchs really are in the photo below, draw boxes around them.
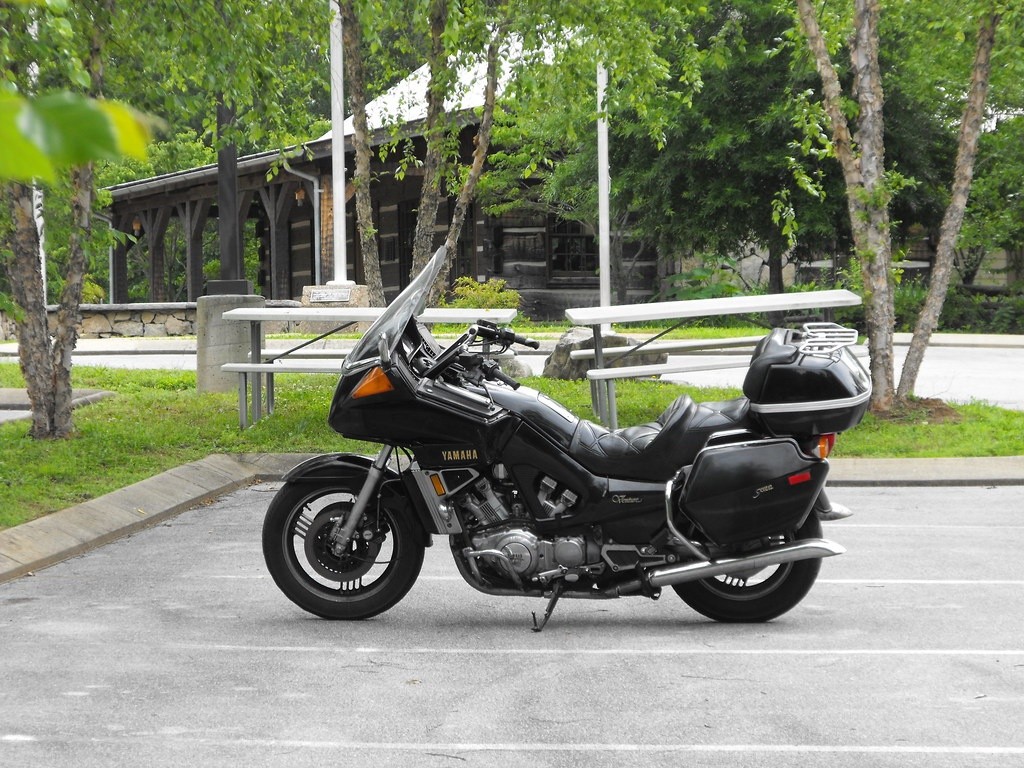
[220,363,343,430]
[571,335,764,416]
[247,349,350,415]
[588,357,754,432]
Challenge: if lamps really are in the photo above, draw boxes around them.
[295,182,305,207]
[133,216,141,236]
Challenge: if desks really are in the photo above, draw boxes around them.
[564,289,862,425]
[223,309,516,423]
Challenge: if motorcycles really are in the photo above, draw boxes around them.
[259,242,875,632]
